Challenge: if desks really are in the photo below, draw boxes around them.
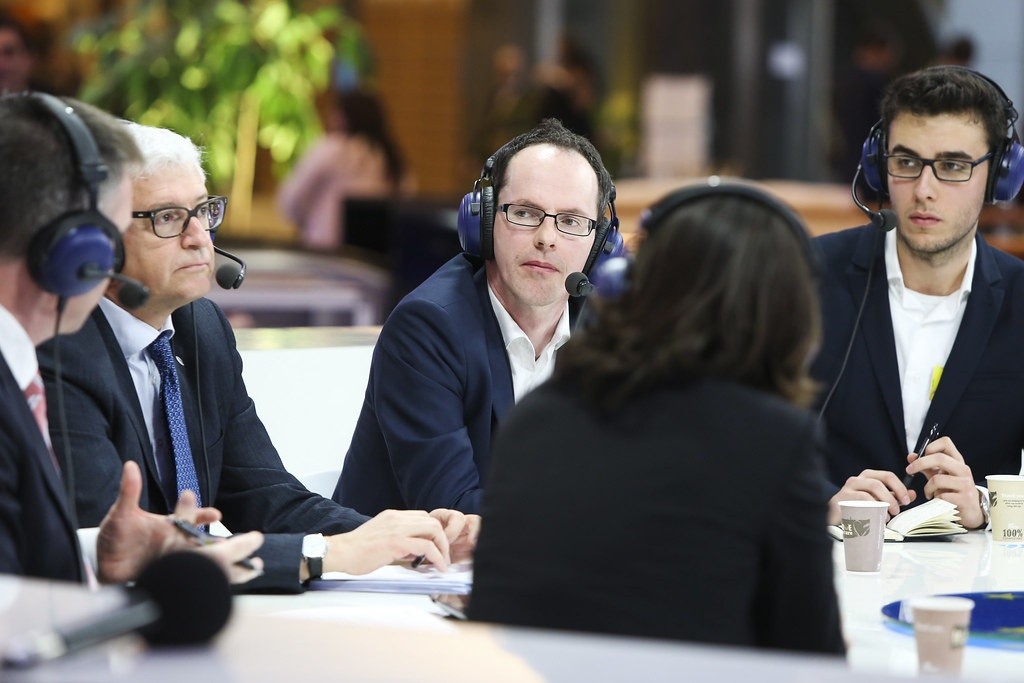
[0,482,1024,683]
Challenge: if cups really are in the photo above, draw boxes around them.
[911,597,974,683]
[985,475,1024,542]
[840,500,891,572]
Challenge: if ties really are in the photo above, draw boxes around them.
[23,370,51,452]
[151,333,205,537]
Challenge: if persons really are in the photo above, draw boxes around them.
[463,180,848,659]
[331,122,627,517]
[484,36,601,164]
[275,84,405,325]
[809,67,1024,525]
[833,20,976,187]
[0,90,264,588]
[0,17,35,94]
[35,111,480,596]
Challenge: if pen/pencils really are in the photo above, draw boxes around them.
[411,555,426,568]
[173,519,254,570]
[903,423,939,490]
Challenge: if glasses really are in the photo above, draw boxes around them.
[495,204,597,237]
[132,195,227,238]
[883,151,994,182]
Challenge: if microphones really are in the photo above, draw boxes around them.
[9,551,236,666]
[564,272,595,298]
[85,271,150,311]
[211,245,247,290]
[851,169,899,232]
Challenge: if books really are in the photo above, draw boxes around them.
[827,498,968,541]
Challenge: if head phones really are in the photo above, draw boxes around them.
[590,177,824,300]
[456,139,629,285]
[18,91,124,301]
[856,65,1024,206]
